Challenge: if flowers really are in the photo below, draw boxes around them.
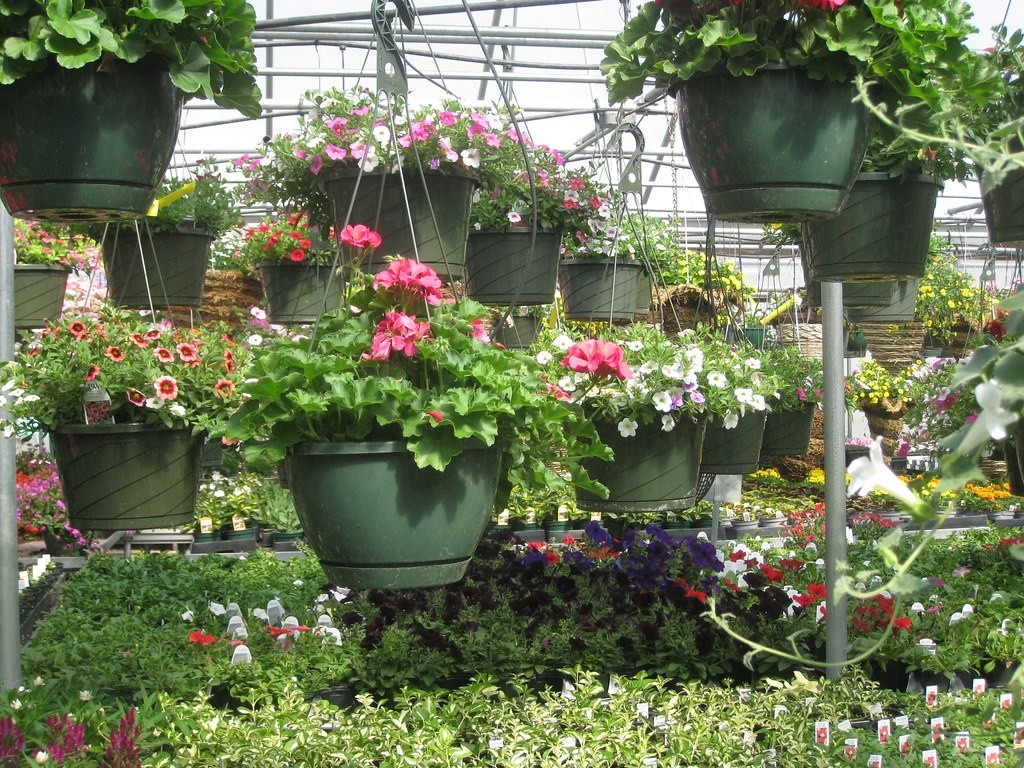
[0,0,1024,768]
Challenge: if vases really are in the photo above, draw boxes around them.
[972,159,1024,248]
[495,315,541,349]
[635,269,651,315]
[842,277,917,323]
[256,261,344,325]
[49,422,209,531]
[557,259,643,324]
[0,54,185,222]
[668,60,873,225]
[14,264,73,330]
[570,406,704,512]
[801,244,891,309]
[95,228,217,309]
[803,174,945,282]
[464,228,563,306]
[702,409,767,475]
[741,329,767,348]
[322,166,481,282]
[844,331,867,357]
[285,441,502,590]
[762,400,816,454]
